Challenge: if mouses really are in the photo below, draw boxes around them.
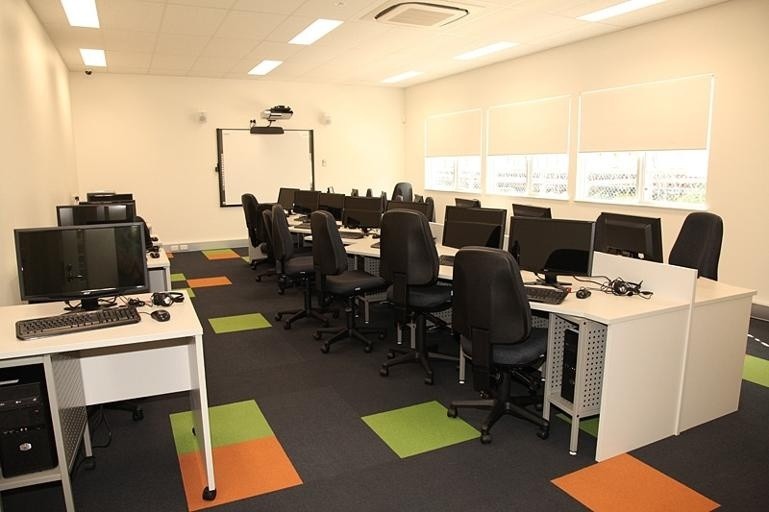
[151,310,170,321]
[150,253,159,258]
[151,237,158,241]
[576,290,591,299]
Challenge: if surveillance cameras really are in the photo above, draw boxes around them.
[84,70,92,75]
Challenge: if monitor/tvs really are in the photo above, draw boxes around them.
[13,222,149,315]
[596,212,663,262]
[508,216,597,286]
[278,186,551,249]
[56,192,136,226]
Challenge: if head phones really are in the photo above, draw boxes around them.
[151,292,184,306]
[614,280,641,294]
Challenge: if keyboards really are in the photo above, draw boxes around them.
[439,255,455,266]
[16,306,141,340]
[524,287,566,304]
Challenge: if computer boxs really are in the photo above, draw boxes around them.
[561,327,579,402]
[0,364,58,479]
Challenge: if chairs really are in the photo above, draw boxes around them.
[669,212,723,286]
[376,209,464,385]
[272,203,339,331]
[446,242,549,443]
[309,209,384,354]
[242,194,305,291]
[365,188,434,221]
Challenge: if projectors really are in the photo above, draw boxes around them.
[261,106,293,120]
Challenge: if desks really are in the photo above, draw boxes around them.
[283,220,696,458]
[681,276,759,432]
[0,286,217,510]
[147,246,174,293]
